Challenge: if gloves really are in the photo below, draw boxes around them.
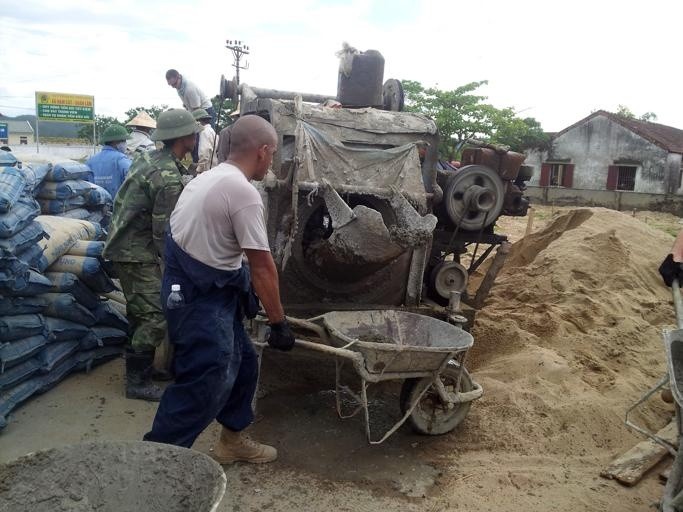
[269,315,295,352]
[659,254,683,288]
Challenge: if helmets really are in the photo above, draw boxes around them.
[151,108,205,141]
[192,109,212,120]
[99,124,133,144]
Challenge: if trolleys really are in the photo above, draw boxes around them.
[246,299,486,449]
[622,273,682,512]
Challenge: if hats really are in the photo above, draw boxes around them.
[125,110,156,128]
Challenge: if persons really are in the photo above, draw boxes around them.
[192,109,220,174]
[166,69,216,164]
[216,100,241,164]
[658,229,683,288]
[85,124,132,212]
[142,114,298,462]
[416,141,465,171]
[100,109,184,402]
[124,111,157,157]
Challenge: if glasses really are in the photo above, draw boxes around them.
[171,77,178,88]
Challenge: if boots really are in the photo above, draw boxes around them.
[125,345,169,402]
[215,425,278,466]
